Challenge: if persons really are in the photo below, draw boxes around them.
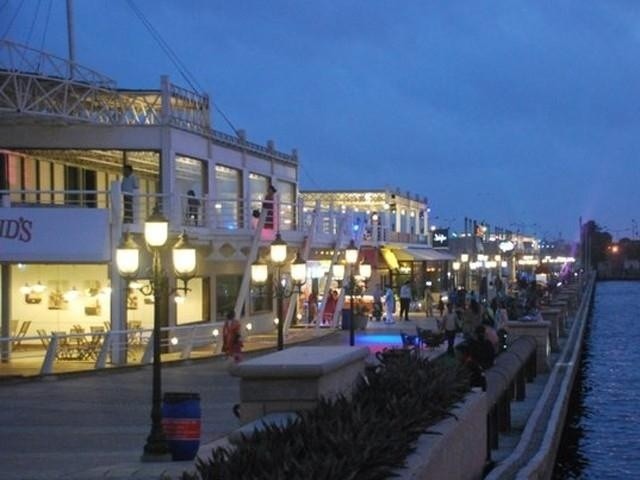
[121,165,141,223]
[304,283,341,328]
[187,188,198,225]
[263,184,277,230]
[371,270,569,392]
[222,310,244,363]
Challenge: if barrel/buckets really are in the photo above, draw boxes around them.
[162,391,203,462]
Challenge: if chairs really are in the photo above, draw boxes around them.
[36,320,143,363]
[10,320,30,352]
[400,325,440,359]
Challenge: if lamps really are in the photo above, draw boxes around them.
[21,281,46,304]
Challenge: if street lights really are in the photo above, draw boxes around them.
[332,237,372,346]
[116,200,198,463]
[251,228,308,350]
[452,249,575,293]
